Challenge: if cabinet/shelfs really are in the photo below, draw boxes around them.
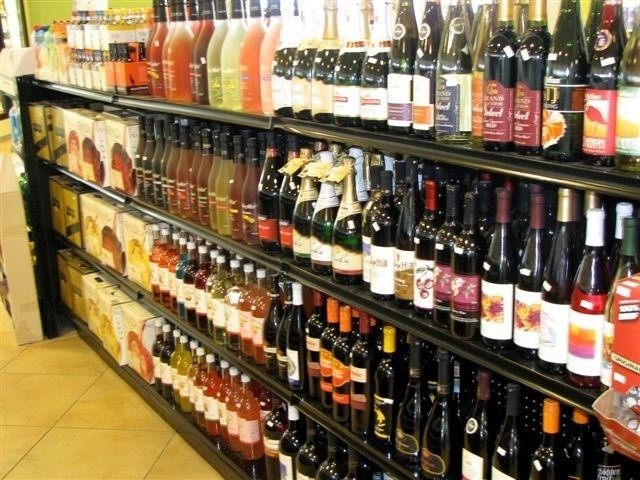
[15,73,640,480]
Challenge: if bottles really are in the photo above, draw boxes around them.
[9,102,23,152]
[134,113,640,478]
[32,0,640,173]
